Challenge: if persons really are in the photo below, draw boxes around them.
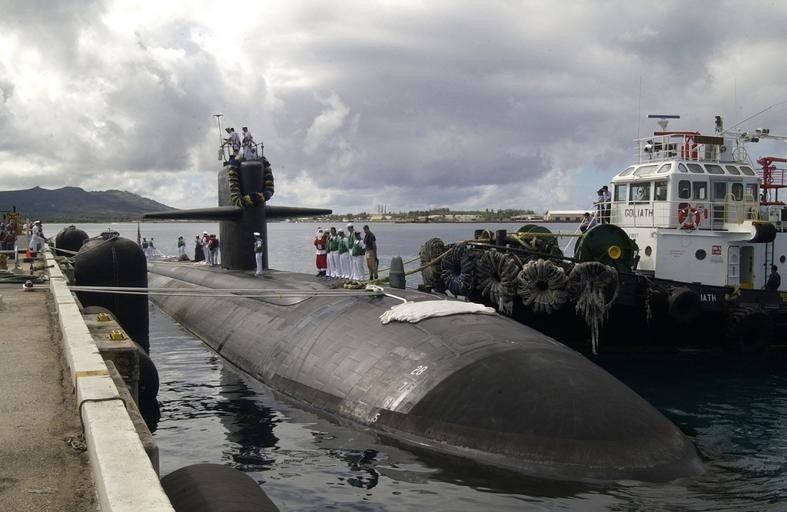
[351,231,366,283]
[314,230,327,276]
[251,231,265,277]
[218,125,256,160]
[600,185,611,222]
[141,237,150,257]
[592,188,605,225]
[1,216,48,266]
[328,227,340,279]
[579,211,597,234]
[346,222,357,281]
[148,237,157,249]
[336,228,351,280]
[362,225,378,281]
[176,231,220,268]
[763,265,782,291]
[322,230,332,277]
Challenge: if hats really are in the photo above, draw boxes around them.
[337,229,344,232]
[347,223,353,228]
[253,232,260,236]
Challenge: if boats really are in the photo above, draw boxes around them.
[417,114,787,386]
